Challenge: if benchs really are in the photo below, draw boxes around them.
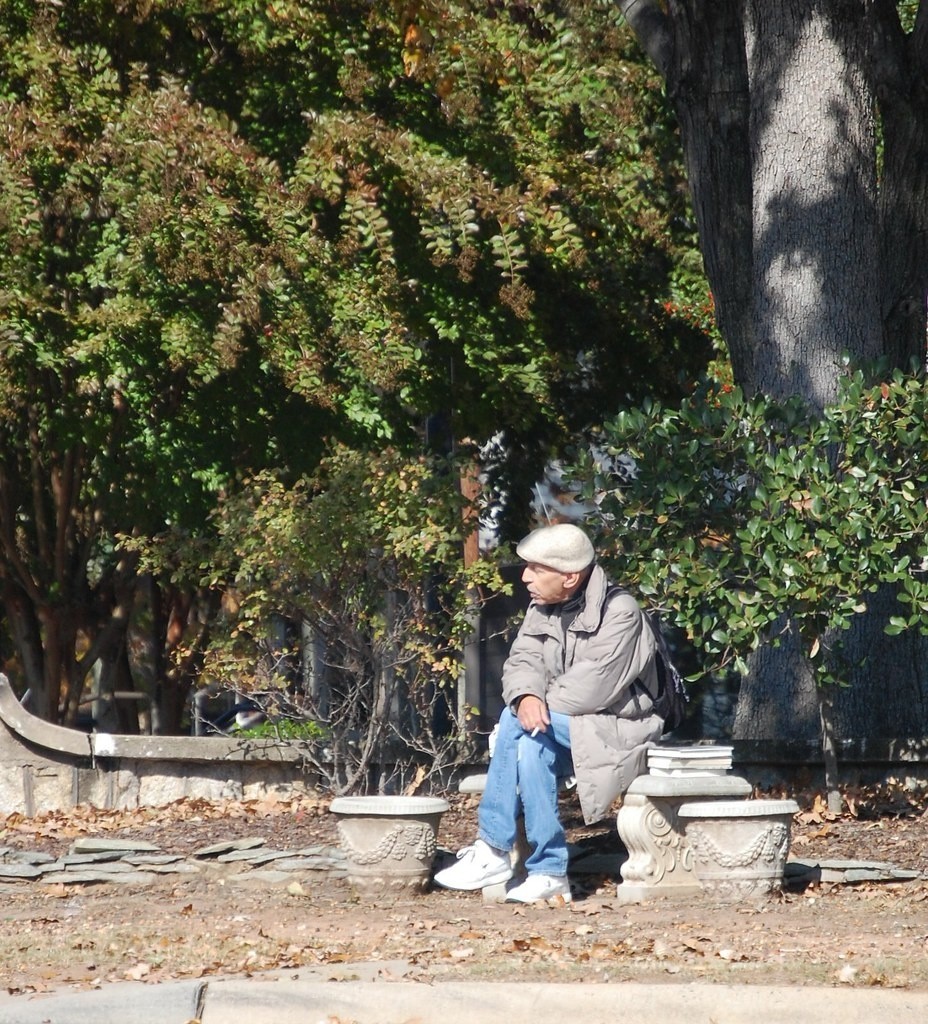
[458,773,752,902]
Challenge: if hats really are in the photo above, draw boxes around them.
[515,523,594,573]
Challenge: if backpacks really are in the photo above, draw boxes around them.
[601,584,687,736]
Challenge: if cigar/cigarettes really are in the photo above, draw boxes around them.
[530,727,540,738]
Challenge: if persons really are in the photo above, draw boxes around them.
[434,524,665,905]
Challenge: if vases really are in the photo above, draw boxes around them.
[677,799,801,901]
[329,796,450,899]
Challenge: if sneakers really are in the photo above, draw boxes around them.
[434,847,513,891]
[505,871,572,904]
